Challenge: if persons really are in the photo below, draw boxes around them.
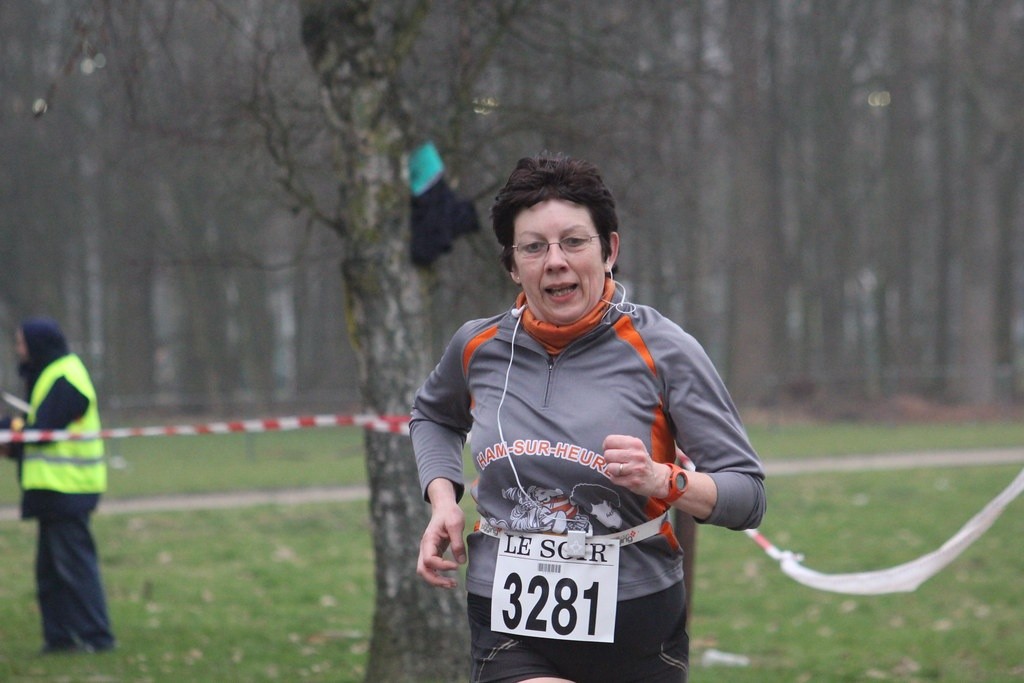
[0,319,116,654]
[408,150,767,683]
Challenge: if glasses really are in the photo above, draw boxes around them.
[512,234,601,262]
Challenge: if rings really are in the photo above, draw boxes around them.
[620,463,623,476]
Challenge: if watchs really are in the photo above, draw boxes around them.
[662,463,689,503]
